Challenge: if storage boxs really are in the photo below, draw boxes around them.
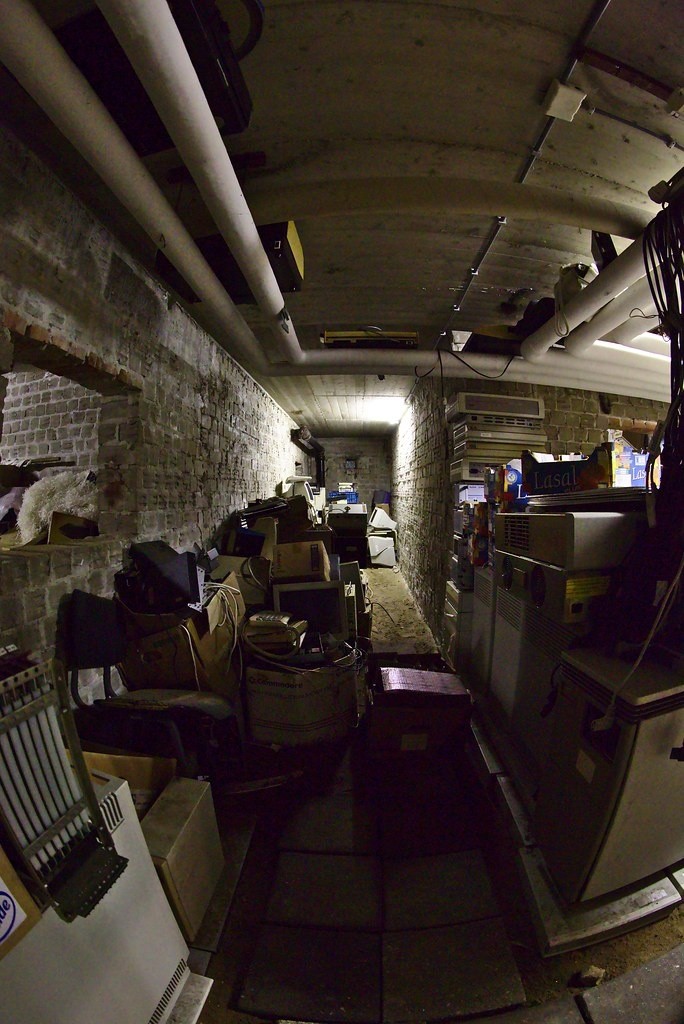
[245,642,359,747]
[272,541,330,582]
[108,571,252,749]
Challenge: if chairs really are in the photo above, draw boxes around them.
[69,590,233,777]
